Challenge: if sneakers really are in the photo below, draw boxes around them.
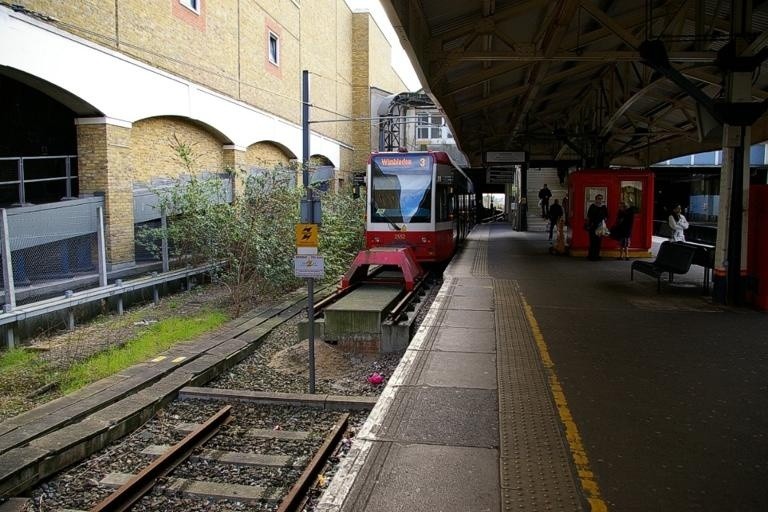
[618,256,624,260]
[626,257,630,261]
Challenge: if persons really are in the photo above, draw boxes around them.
[539,184,553,218]
[561,192,569,221]
[586,193,608,262]
[547,198,563,240]
[668,202,690,243]
[614,200,634,260]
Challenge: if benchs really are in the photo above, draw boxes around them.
[630,242,697,294]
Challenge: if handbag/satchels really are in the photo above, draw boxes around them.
[583,223,588,230]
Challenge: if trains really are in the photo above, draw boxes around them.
[365,145,476,262]
